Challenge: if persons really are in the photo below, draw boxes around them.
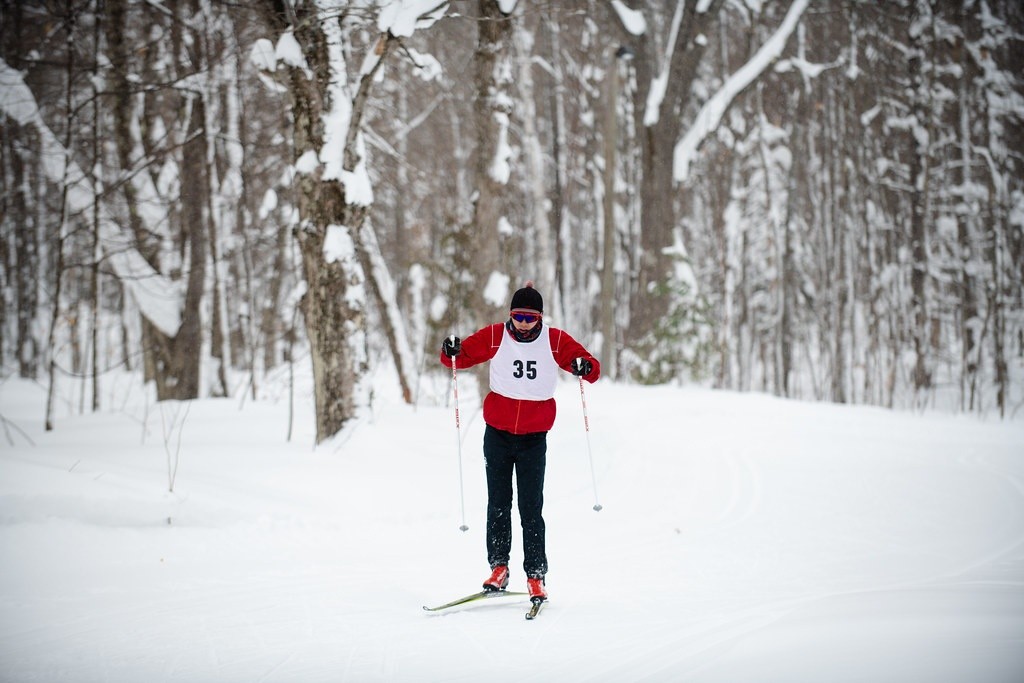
[440,282,599,607]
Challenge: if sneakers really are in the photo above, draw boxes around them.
[527,578,549,602]
[483,565,509,590]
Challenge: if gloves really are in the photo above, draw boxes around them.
[570,357,592,376]
[442,336,461,357]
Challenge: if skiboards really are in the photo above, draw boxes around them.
[422,589,544,621]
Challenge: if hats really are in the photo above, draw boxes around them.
[508,280,543,318]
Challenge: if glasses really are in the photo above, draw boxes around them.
[511,313,538,323]
